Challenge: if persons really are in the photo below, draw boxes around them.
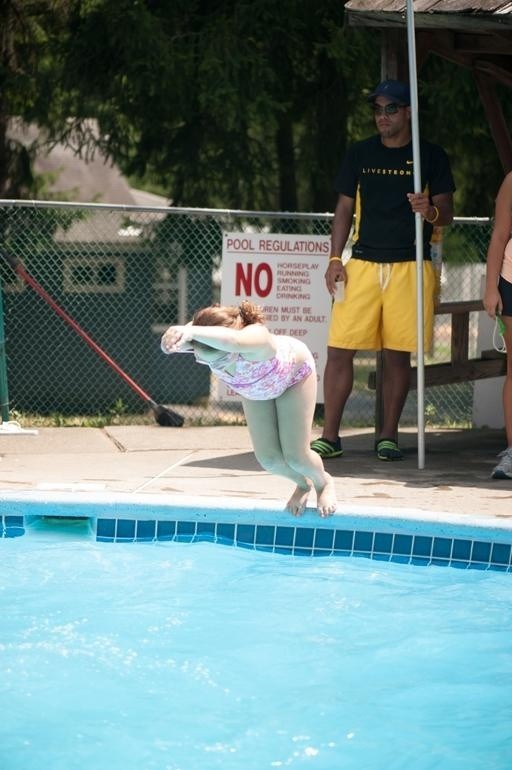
[480,169,510,480]
[158,298,340,518]
[309,77,460,462]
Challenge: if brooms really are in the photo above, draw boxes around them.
[15,262,185,428]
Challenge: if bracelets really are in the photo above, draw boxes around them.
[325,255,343,263]
[427,204,442,226]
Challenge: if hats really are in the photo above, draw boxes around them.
[368,80,410,106]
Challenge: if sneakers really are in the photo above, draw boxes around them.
[375,439,402,461]
[310,437,344,458]
[490,447,512,479]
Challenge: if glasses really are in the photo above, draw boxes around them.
[368,104,407,114]
